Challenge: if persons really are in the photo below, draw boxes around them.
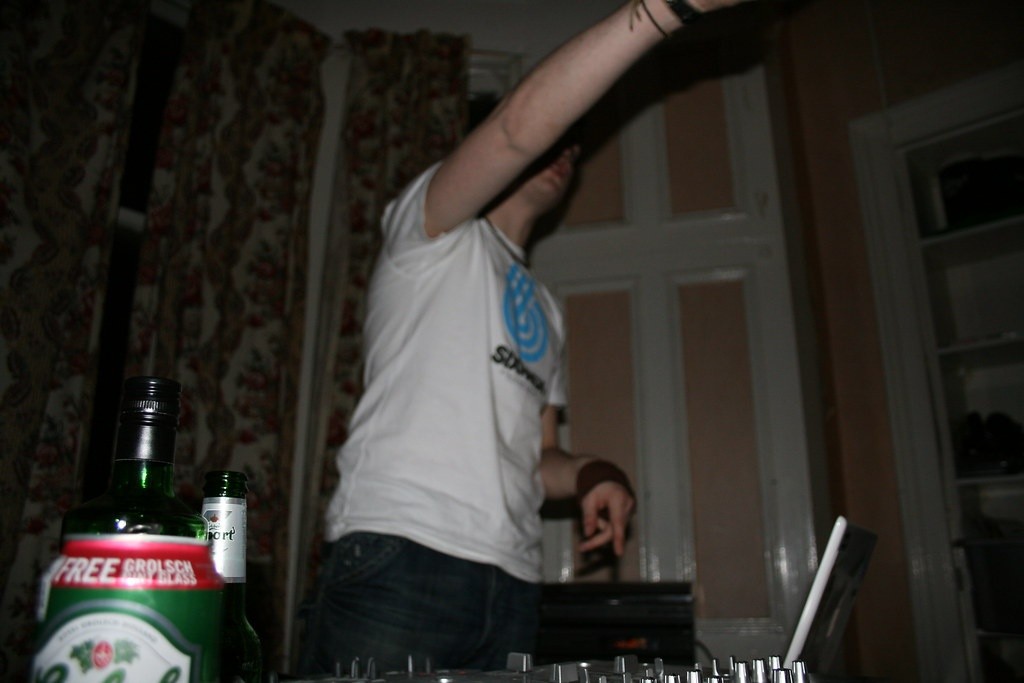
[291,0,739,683]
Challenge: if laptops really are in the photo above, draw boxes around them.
[782,517,881,677]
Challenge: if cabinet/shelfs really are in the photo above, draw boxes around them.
[892,105,1024,683]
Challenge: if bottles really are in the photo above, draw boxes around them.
[32,375,224,683]
[201,470,263,683]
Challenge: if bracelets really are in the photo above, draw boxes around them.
[629,0,668,38]
[576,458,637,501]
[664,0,705,29]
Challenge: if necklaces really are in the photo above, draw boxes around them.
[481,212,527,268]
